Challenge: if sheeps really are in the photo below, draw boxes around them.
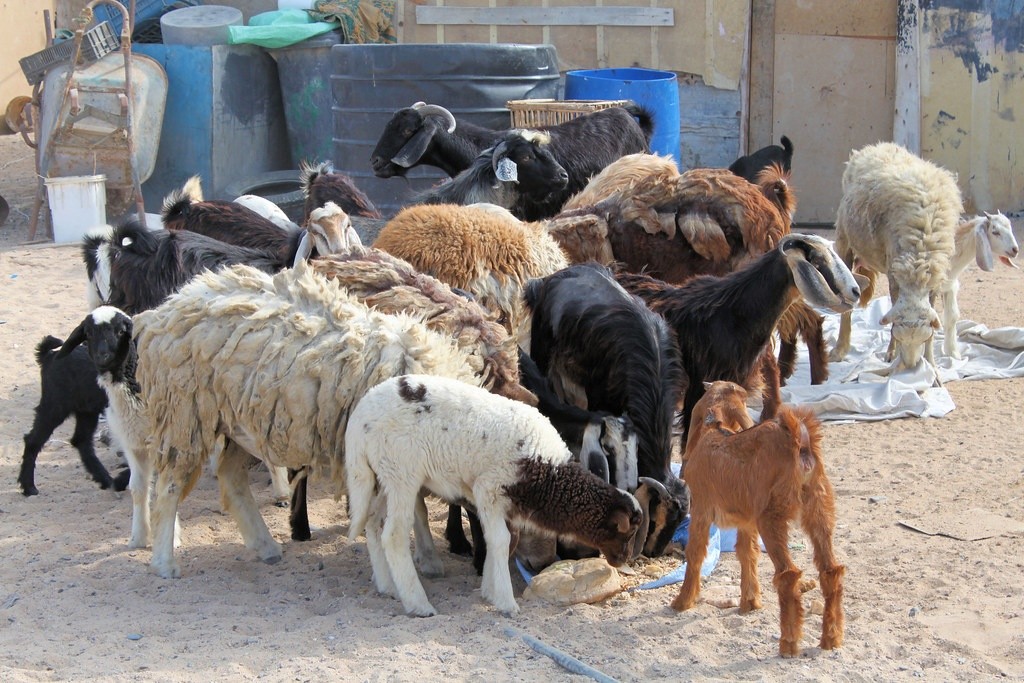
[675,376,849,657]
[15,97,861,613]
[828,140,1020,374]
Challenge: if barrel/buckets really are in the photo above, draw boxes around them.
[43,173,108,246]
[565,69,681,174]
